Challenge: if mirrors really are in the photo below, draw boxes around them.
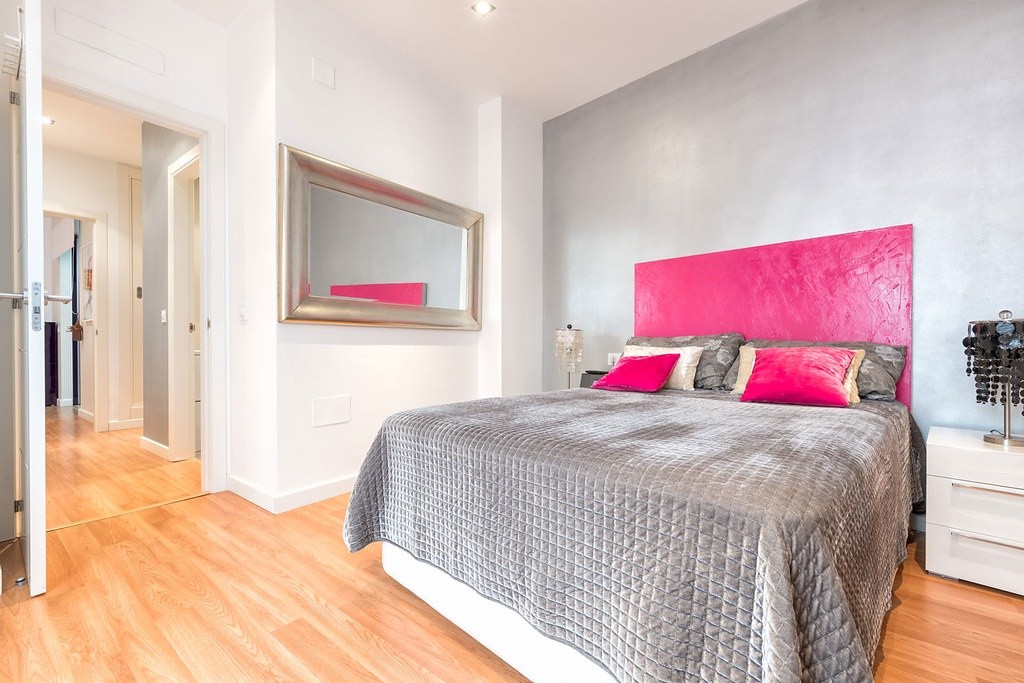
[277,142,484,332]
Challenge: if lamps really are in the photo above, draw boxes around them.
[555,324,584,389]
[963,310,1024,447]
[342,224,926,683]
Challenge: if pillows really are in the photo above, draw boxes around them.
[590,353,681,393]
[729,346,866,404]
[720,337,906,402]
[624,345,705,391]
[626,332,746,390]
[740,347,856,408]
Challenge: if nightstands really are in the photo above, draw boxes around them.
[926,426,1024,596]
